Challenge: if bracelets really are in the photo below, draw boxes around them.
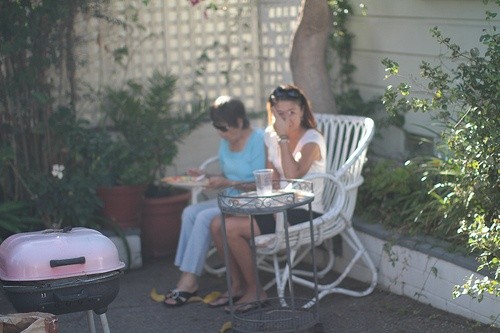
[278,135,290,143]
[232,180,236,190]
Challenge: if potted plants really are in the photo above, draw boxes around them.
[49,72,212,265]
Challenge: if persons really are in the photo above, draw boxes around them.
[208,85,327,313]
[158,95,268,307]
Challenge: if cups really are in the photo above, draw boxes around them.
[252,168,274,200]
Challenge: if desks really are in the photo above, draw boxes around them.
[217,179,319,333]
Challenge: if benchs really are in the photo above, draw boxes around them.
[191,114,377,312]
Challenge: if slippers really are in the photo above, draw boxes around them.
[161,290,202,306]
[221,298,267,313]
[208,291,242,307]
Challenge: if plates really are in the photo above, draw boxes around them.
[160,175,211,188]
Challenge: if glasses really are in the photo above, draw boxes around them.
[215,120,237,131]
[273,90,302,100]
